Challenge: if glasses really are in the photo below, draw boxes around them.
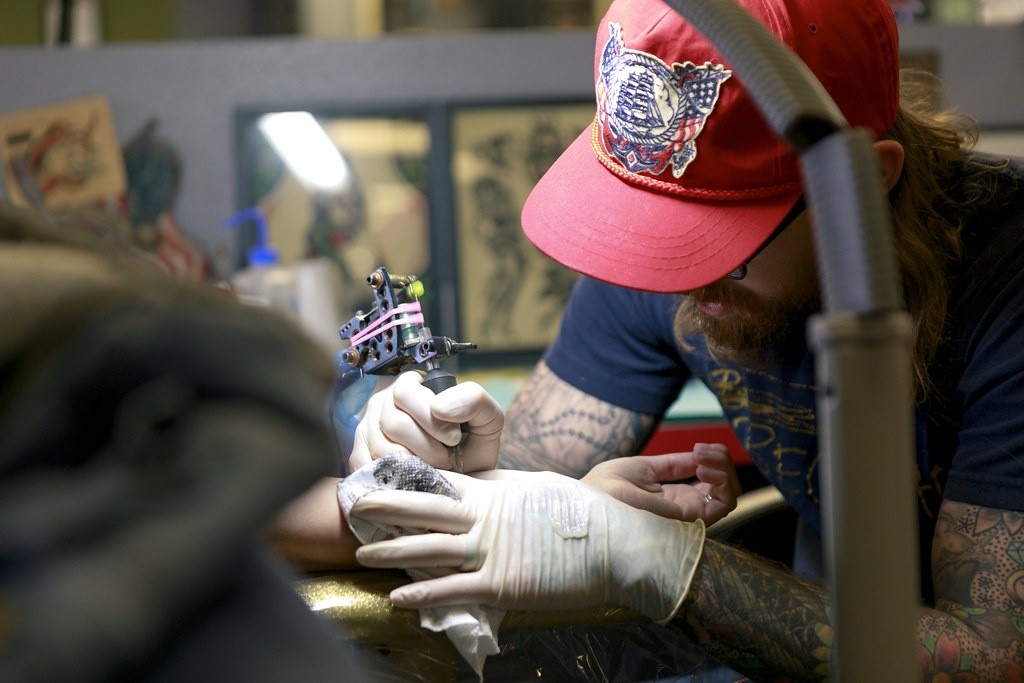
[725,192,808,281]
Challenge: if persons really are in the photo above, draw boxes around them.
[347,0,1024,683]
[265,444,739,567]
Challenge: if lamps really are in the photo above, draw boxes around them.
[257,105,368,251]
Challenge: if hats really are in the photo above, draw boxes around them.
[519,1,900,300]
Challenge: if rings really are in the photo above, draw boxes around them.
[705,493,713,503]
